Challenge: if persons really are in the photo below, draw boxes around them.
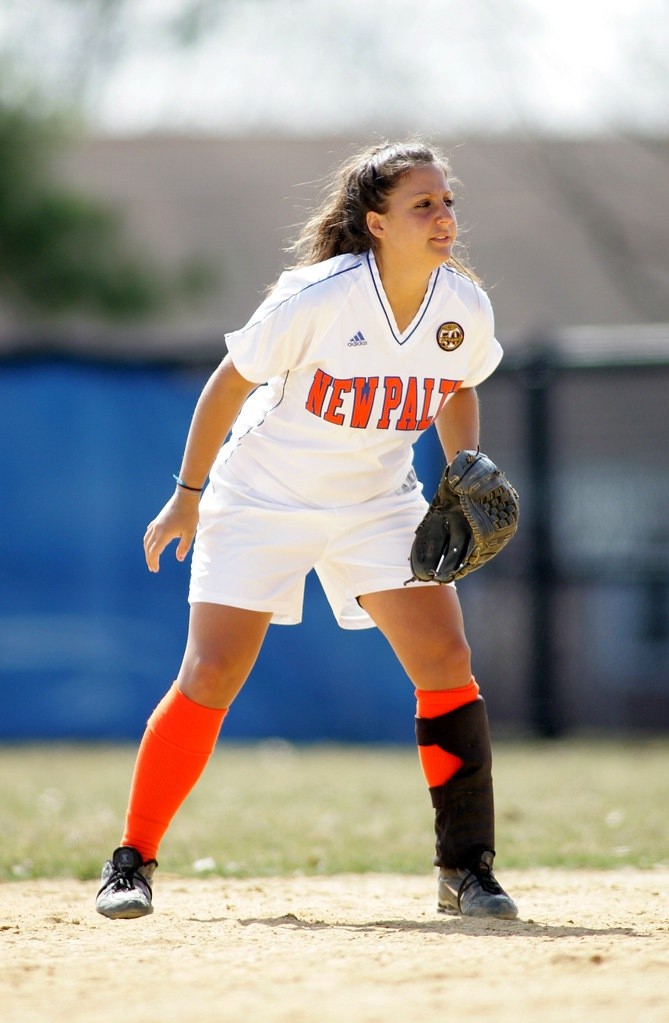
[94,136,521,922]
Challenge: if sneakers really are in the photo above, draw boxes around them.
[96,845,158,919]
[437,848,519,920]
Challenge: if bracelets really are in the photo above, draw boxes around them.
[173,474,203,492]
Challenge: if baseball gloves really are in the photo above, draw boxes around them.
[410,450,521,587]
